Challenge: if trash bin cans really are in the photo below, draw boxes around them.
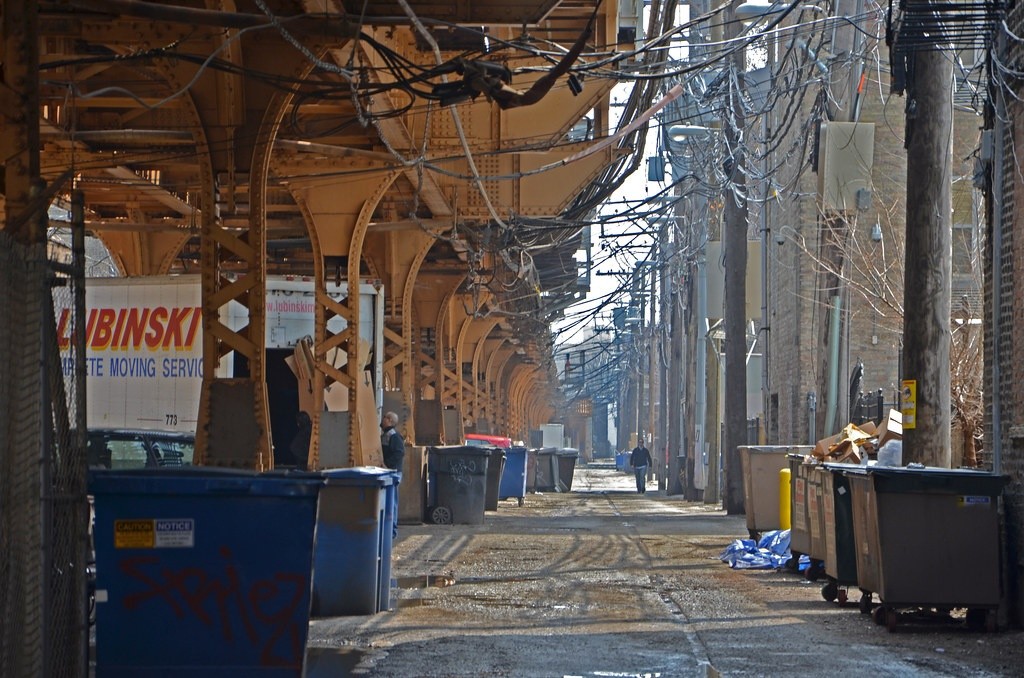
[78,461,334,678]
[615,452,634,474]
[531,447,580,493]
[481,446,507,512]
[310,464,404,619]
[783,451,1014,635]
[736,444,818,540]
[499,447,528,508]
[428,445,493,525]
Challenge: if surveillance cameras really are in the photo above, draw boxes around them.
[775,235,785,245]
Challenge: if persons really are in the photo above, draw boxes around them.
[630,437,653,494]
[381,411,405,538]
[291,409,312,466]
